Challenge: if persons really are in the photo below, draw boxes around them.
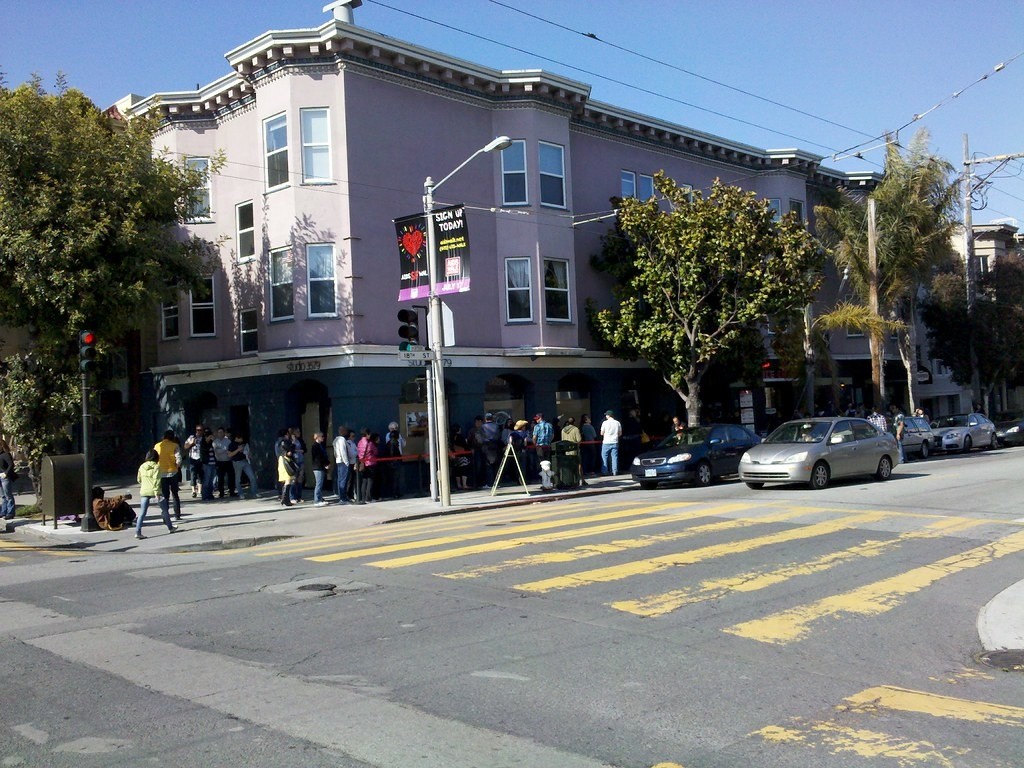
[185,409,741,507]
[92,486,137,531]
[153,430,187,524]
[792,399,986,463]
[134,449,179,539]
[0,439,15,520]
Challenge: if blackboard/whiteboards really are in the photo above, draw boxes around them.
[492,444,527,491]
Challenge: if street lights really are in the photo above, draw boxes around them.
[425,135,512,507]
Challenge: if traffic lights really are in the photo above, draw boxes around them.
[80,330,97,373]
[397,308,419,352]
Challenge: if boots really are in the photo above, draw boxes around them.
[281,484,294,507]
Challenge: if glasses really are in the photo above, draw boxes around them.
[322,435,324,438]
[196,429,202,431]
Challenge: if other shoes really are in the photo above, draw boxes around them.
[239,495,245,499]
[314,500,328,507]
[134,534,147,539]
[192,492,197,497]
[170,524,178,533]
[254,493,262,497]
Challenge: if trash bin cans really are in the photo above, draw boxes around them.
[551,440,582,489]
[41,453,84,517]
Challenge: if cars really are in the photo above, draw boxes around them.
[929,412,998,454]
[738,417,898,490]
[630,424,762,490]
[890,417,934,460]
[990,409,1024,447]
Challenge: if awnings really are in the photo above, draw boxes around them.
[848,358,933,389]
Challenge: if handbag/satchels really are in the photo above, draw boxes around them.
[354,461,365,472]
[284,460,300,475]
[454,445,465,452]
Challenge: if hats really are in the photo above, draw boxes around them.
[514,419,529,431]
[604,410,614,415]
